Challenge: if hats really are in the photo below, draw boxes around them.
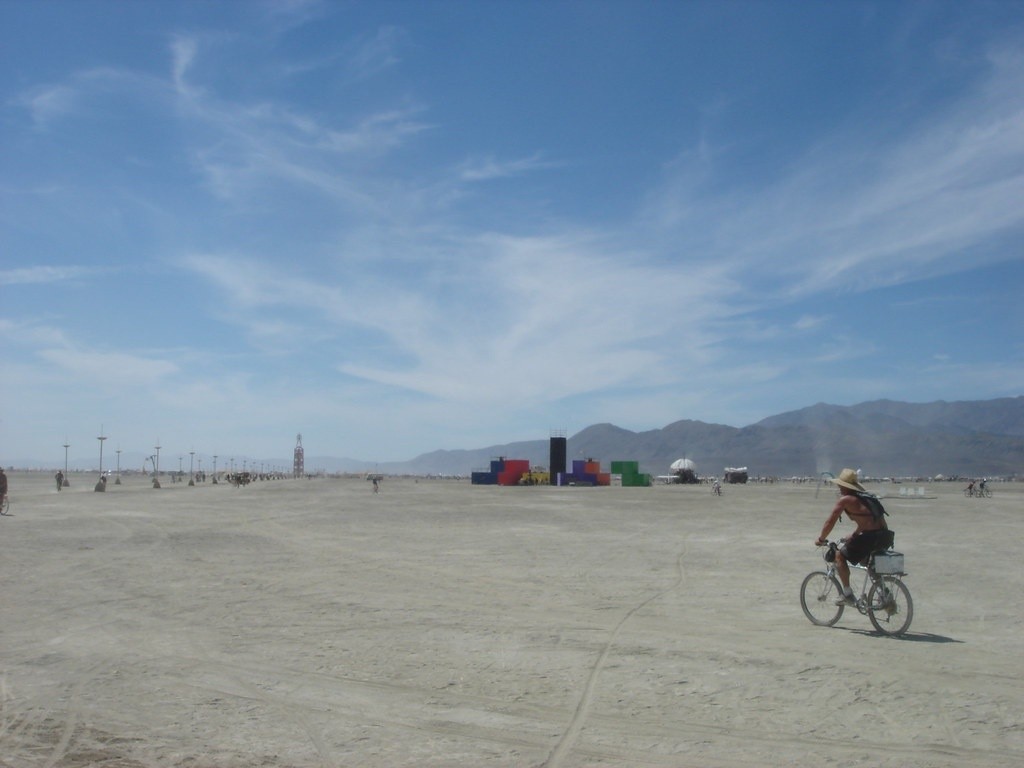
[829,469,867,493]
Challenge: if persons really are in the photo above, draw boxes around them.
[964,479,975,498]
[979,478,987,498]
[55,470,63,491]
[0,466,8,513]
[101,470,106,489]
[227,470,287,486]
[712,477,721,495]
[195,469,205,482]
[813,468,892,606]
[372,473,380,495]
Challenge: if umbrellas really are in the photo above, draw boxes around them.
[671,458,697,472]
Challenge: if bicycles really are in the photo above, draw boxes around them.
[0,495,10,516]
[964,485,994,499]
[800,538,915,639]
[711,485,724,496]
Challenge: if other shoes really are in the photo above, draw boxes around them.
[834,593,856,606]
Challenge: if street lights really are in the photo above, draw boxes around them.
[63,444,71,487]
[150,446,164,489]
[114,450,122,484]
[189,452,195,486]
[197,455,277,485]
[96,436,106,492]
[178,457,183,482]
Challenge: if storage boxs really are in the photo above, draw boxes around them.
[875,550,905,574]
